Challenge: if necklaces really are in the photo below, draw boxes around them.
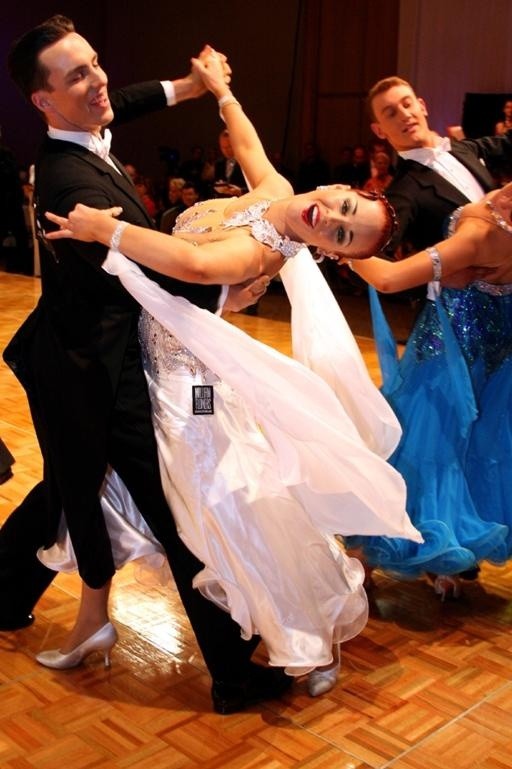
[220,197,306,259]
[486,198,512,233]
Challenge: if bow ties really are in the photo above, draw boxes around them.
[413,138,454,164]
[88,128,114,158]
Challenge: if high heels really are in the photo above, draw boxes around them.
[35,619,118,673]
[307,624,342,697]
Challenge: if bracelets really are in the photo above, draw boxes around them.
[109,219,128,253]
[217,95,237,107]
[346,257,355,271]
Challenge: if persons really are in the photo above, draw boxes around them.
[36,46,424,713]
[336,181,512,603]
[365,74,512,343]
[0,13,294,716]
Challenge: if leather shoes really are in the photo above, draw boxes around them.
[0,612,36,632]
[434,570,462,603]
[212,661,296,713]
